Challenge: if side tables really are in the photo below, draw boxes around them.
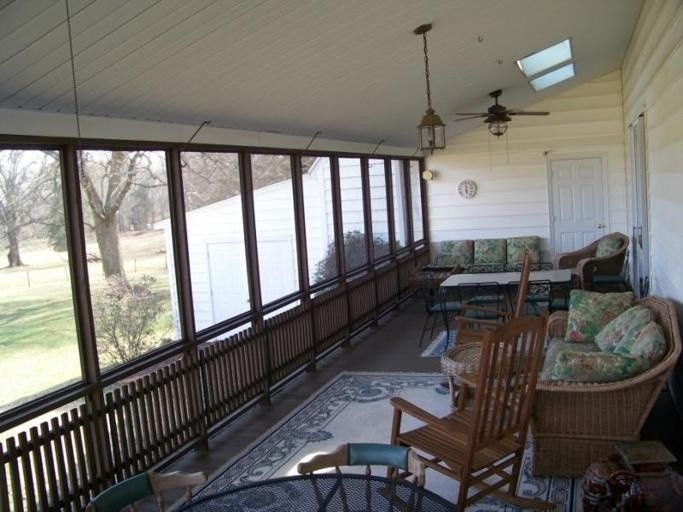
[440,346,524,413]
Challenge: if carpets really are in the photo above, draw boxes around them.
[161,368,585,511]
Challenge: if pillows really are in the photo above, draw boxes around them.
[551,287,666,383]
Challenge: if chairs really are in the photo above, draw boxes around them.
[418,259,553,349]
[376,312,550,510]
[418,232,629,351]
[84,469,207,512]
[455,252,530,344]
[297,441,424,510]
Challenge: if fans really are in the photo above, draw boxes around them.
[453,90,550,140]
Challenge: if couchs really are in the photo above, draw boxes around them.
[408,235,541,291]
[529,294,682,477]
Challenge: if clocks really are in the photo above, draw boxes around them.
[457,179,477,200]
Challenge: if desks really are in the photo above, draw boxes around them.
[442,268,571,316]
[177,472,460,512]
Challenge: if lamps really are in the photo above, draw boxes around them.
[413,24,445,156]
